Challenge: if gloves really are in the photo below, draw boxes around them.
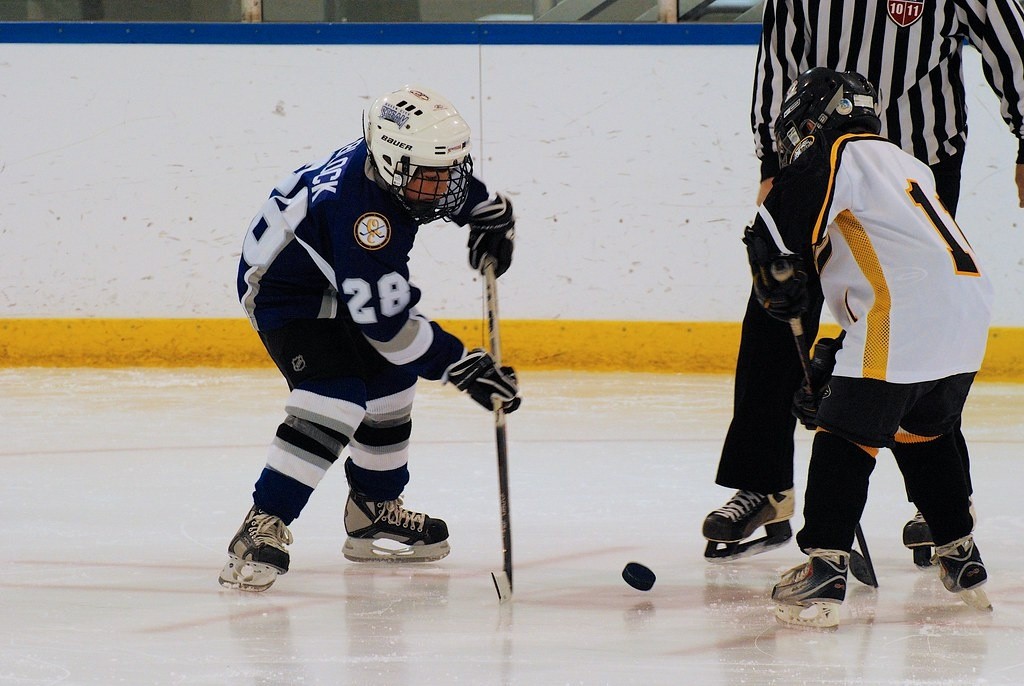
[790,337,843,430]
[444,348,520,413]
[468,191,515,279]
[743,237,809,324]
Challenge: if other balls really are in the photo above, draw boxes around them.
[622,562,656,595]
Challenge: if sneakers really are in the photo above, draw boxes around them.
[903,496,976,565]
[939,543,995,614]
[771,556,849,627]
[703,482,796,563]
[342,488,451,563]
[219,505,293,592]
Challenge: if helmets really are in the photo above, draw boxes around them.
[368,85,473,189]
[774,67,879,149]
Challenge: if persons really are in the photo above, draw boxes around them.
[742,66,990,606]
[702,1,1024,548]
[223,86,523,576]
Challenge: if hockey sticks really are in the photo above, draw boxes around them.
[480,250,515,600]
[768,258,880,590]
